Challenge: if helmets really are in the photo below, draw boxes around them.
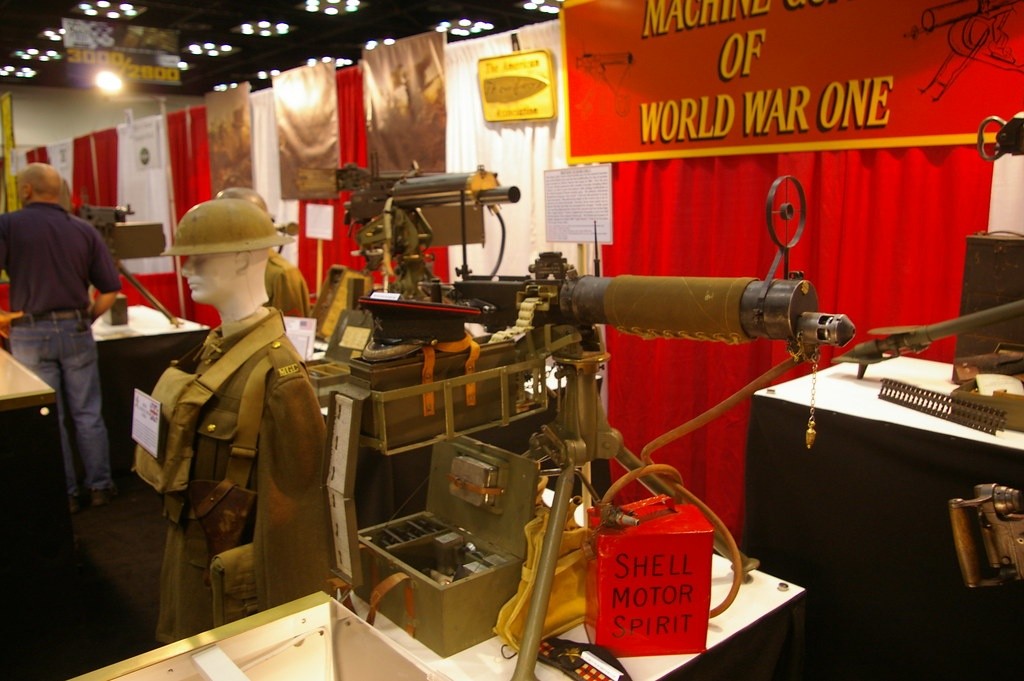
[160,199,296,257]
[214,187,275,220]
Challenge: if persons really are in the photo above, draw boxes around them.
[156,186,332,640]
[0,161,118,511]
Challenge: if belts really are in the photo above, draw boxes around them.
[11,309,89,325]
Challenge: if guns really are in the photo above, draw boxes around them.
[334,162,520,274]
[78,203,136,228]
[445,173,856,349]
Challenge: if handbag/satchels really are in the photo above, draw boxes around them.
[132,366,213,494]
[211,542,261,629]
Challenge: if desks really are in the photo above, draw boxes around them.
[740,352,1024,681]
[333,485,806,681]
[90,303,210,473]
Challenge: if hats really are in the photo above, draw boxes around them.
[358,297,481,363]
